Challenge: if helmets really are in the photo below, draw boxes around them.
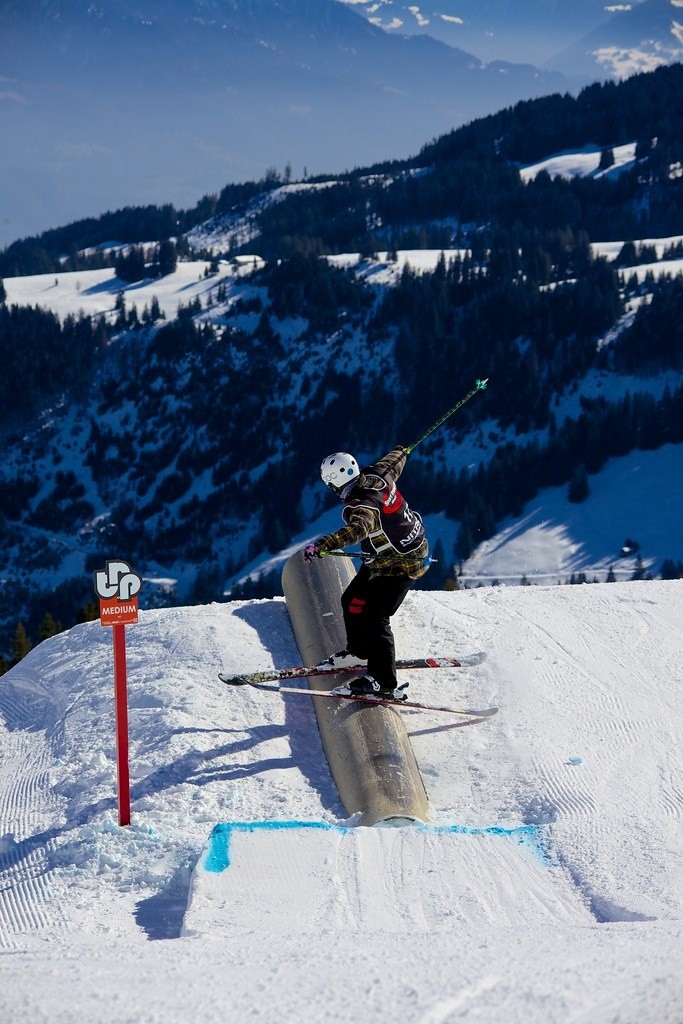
[320,452,360,488]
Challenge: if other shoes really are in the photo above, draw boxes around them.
[328,650,368,667]
[349,675,394,699]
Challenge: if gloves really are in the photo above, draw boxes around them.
[392,445,410,459]
[303,544,323,564]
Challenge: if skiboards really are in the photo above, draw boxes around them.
[218,651,500,719]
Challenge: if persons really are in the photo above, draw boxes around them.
[303,442,432,694]
[115,290,125,310]
